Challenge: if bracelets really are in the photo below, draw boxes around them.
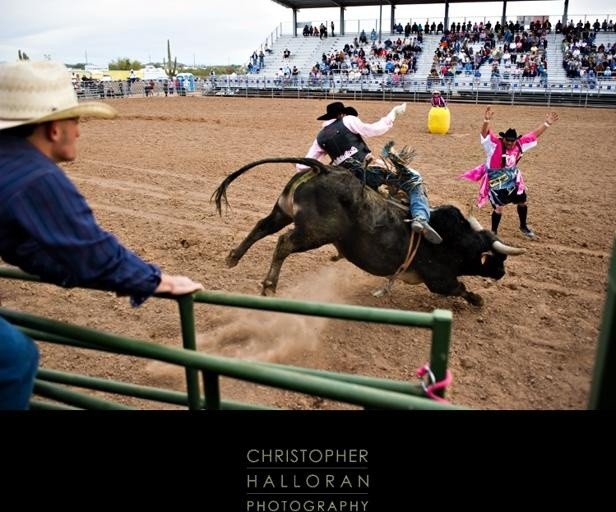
[545,122,550,129]
[484,119,490,123]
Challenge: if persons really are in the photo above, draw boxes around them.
[1,58,209,412]
[479,104,559,237]
[71,65,236,98]
[295,100,443,245]
[246,13,616,98]
[431,91,446,107]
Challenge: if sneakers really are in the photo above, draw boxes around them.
[519,225,534,237]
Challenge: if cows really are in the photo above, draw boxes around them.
[210,156,527,308]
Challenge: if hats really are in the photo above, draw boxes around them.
[0,59,118,132]
[432,90,441,96]
[500,128,522,139]
[317,102,358,121]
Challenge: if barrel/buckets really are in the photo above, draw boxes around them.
[428,105,451,135]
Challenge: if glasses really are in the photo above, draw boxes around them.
[506,138,515,142]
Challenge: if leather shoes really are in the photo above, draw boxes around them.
[411,215,443,244]
